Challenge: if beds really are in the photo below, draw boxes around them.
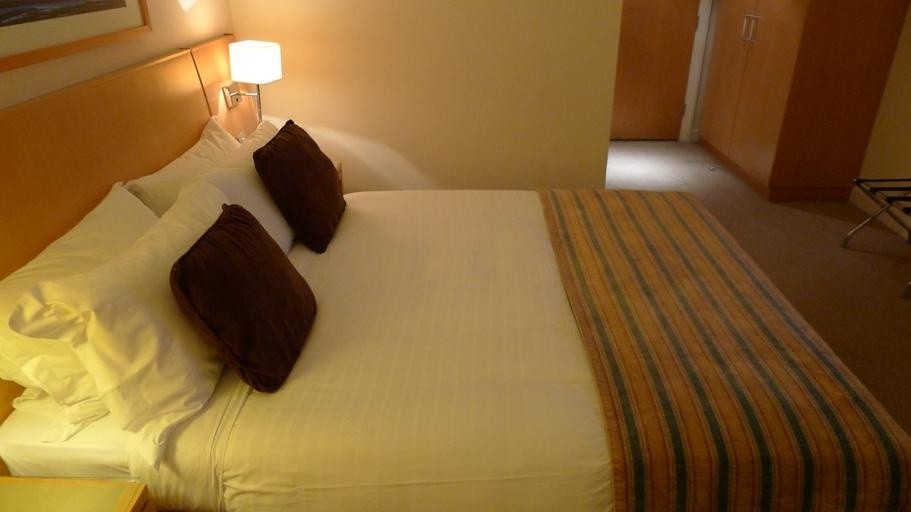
[3,191,911,512]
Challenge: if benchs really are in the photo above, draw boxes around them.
[842,176,911,299]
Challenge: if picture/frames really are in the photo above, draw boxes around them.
[0,0,154,74]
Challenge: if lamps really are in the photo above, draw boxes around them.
[221,40,283,124]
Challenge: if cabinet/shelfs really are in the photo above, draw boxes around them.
[695,1,911,205]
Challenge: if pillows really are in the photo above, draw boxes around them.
[7,177,227,472]
[169,201,322,395]
[178,119,295,256]
[121,115,241,213]
[3,173,162,444]
[251,116,343,257]
